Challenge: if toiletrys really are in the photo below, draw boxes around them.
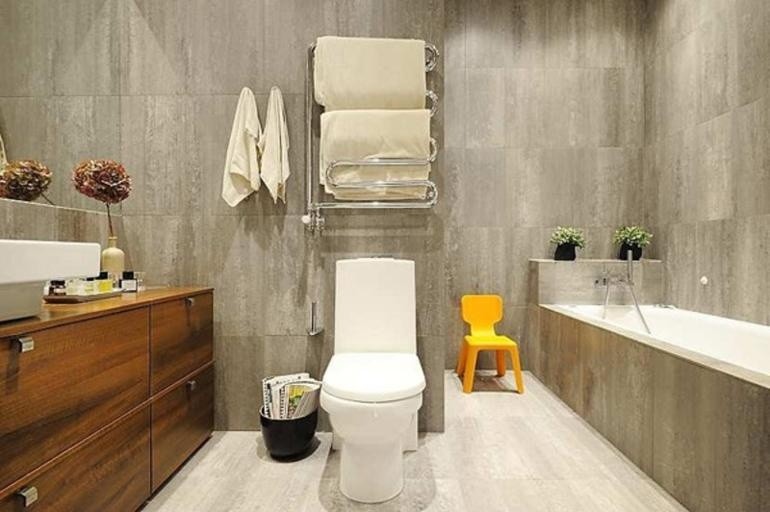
[48,271,138,296]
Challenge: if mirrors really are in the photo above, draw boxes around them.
[0,0,124,218]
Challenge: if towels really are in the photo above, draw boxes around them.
[257,86,293,207]
[220,86,264,208]
[311,33,431,112]
[317,109,430,205]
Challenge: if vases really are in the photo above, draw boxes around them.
[98,235,128,274]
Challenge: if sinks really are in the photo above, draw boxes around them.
[0,239,101,323]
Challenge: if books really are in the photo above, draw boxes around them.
[262,372,323,420]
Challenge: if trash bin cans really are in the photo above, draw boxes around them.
[259,405,318,458]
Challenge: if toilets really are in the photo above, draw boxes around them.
[318,256,427,504]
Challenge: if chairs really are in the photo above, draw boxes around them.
[451,292,527,394]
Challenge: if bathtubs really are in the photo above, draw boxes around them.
[540,303,769,511]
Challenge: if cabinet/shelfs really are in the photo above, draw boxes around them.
[149,291,217,496]
[1,304,154,509]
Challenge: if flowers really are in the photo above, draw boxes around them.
[1,159,55,204]
[73,153,133,235]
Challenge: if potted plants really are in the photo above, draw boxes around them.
[610,223,651,259]
[550,224,585,261]
